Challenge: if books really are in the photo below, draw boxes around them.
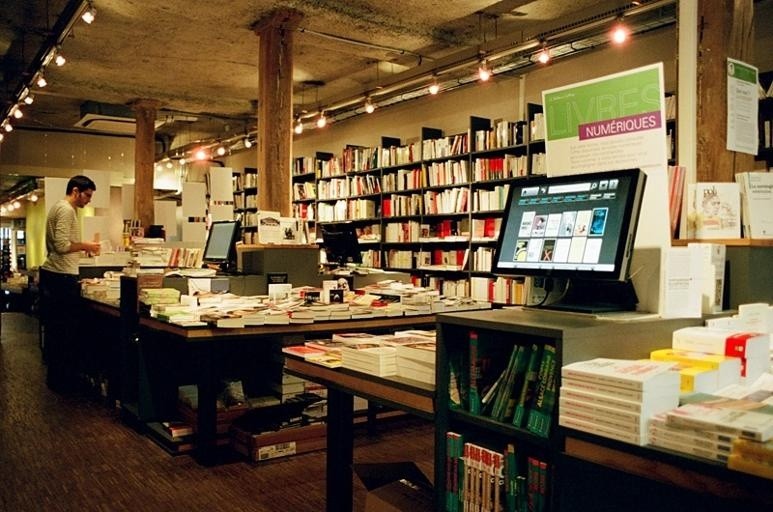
[656,165,772,318]
[231,110,551,305]
[557,302,772,478]
[444,328,561,512]
[282,329,439,386]
[161,368,369,439]
[78,217,444,329]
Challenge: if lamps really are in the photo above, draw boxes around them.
[242,135,258,149]
[216,138,225,157]
[0,6,99,144]
[364,93,378,114]
[316,110,328,128]
[196,145,206,161]
[537,41,551,65]
[608,11,632,48]
[478,56,492,83]
[292,110,307,135]
[428,74,438,95]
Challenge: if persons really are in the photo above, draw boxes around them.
[39,175,102,392]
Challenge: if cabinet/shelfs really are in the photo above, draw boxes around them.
[189,92,675,305]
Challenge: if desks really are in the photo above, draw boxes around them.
[282,331,772,512]
[75,293,505,467]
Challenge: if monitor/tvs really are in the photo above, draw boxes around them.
[320,220,363,267]
[202,221,240,274]
[491,165,648,313]
